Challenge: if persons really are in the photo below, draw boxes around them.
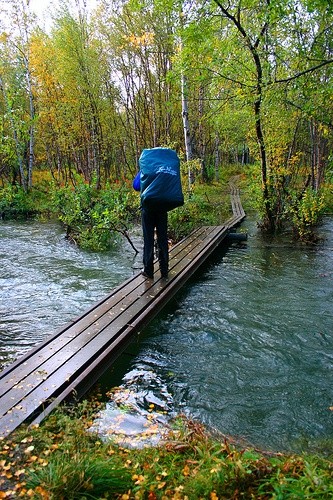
[132,168,170,282]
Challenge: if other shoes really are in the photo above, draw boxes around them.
[140,269,155,279]
[160,269,168,276]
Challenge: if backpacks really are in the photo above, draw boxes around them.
[139,147,184,214]
